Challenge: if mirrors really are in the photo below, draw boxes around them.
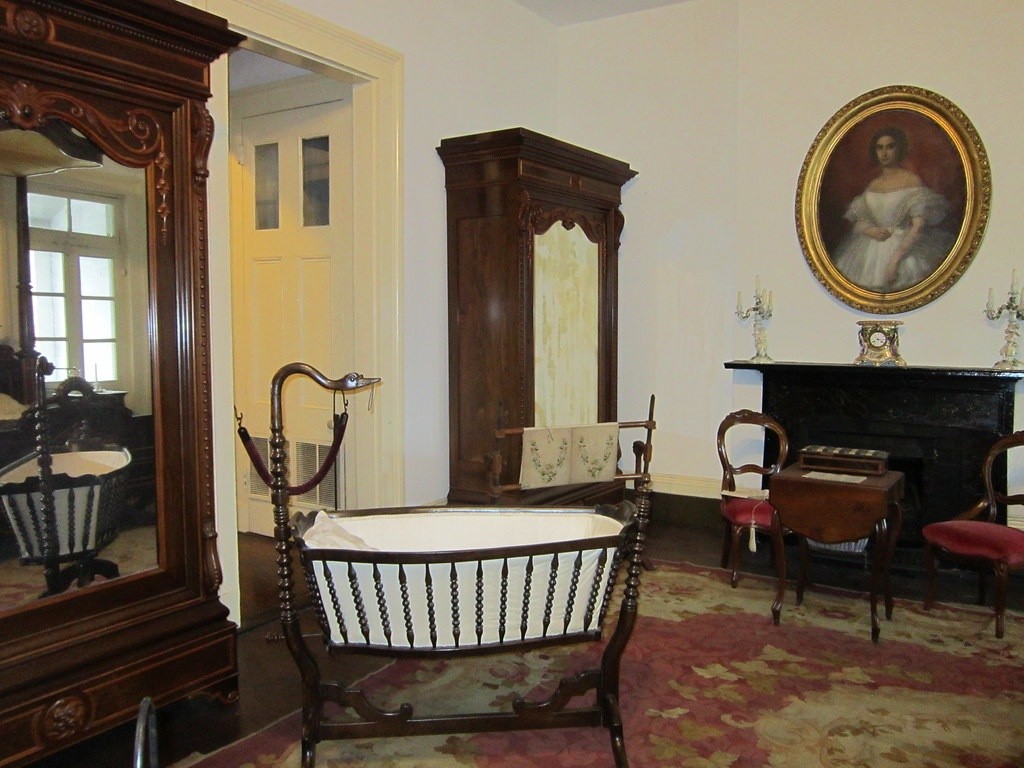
[527,203,607,428]
[1,74,198,644]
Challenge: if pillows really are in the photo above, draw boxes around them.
[306,510,368,550]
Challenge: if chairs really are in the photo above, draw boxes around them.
[715,408,813,588]
[921,431,1024,640]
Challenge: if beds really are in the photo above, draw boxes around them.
[239,359,657,767]
[0,352,131,600]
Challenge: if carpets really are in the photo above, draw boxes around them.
[180,554,1024,768]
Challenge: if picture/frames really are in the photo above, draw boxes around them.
[794,84,994,314]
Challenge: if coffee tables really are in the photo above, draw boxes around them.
[768,461,902,641]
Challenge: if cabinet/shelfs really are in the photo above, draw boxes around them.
[433,127,657,576]
[0,1,250,768]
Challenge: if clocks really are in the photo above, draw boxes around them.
[855,320,906,367]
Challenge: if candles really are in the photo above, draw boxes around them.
[981,268,1024,314]
[734,274,776,313]
[94,362,98,382]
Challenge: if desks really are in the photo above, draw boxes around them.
[725,359,1024,605]
[53,388,130,408]
